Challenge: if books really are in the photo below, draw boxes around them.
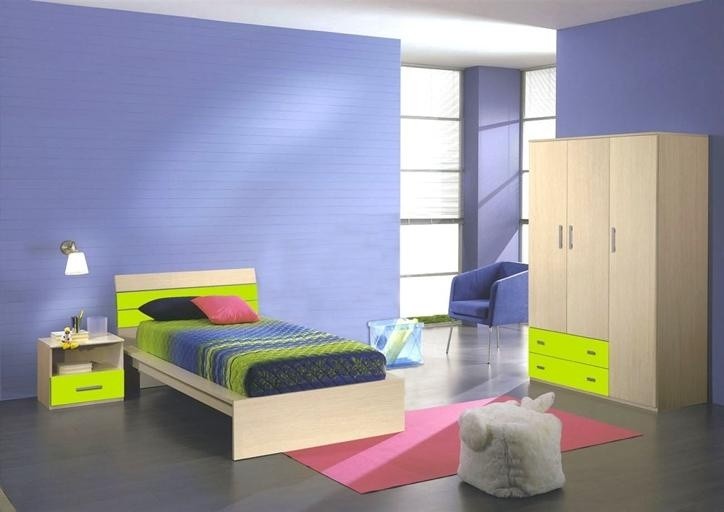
[50,329,88,339]
[57,361,92,374]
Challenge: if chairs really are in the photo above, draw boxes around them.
[446,262,529,364]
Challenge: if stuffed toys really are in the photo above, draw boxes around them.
[61,327,80,349]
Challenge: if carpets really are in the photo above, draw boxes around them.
[284,396,645,494]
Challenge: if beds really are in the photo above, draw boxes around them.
[114,267,406,461]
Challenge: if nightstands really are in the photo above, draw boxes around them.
[36,331,125,410]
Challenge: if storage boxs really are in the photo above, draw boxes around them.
[368,320,425,369]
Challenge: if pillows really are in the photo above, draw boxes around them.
[138,297,207,320]
[191,295,261,325]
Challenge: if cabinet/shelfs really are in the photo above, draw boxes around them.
[529,132,709,412]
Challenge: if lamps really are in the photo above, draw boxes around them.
[61,240,89,275]
